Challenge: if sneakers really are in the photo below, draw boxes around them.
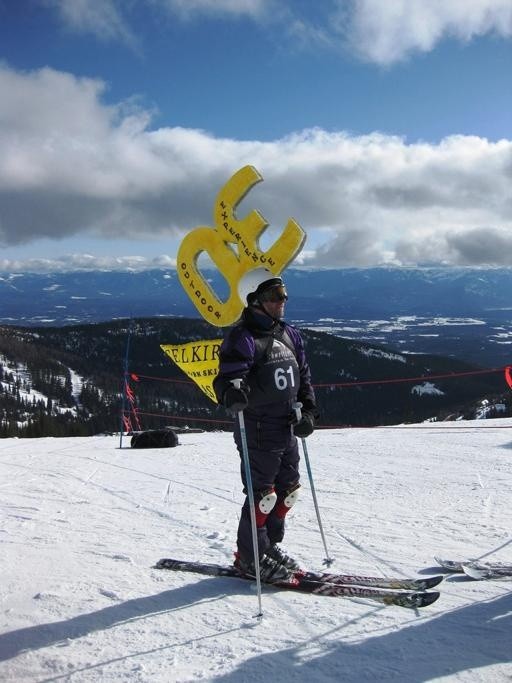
[233,543,300,577]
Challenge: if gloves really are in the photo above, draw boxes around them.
[221,386,249,414]
[290,409,315,437]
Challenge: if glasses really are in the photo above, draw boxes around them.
[261,284,288,303]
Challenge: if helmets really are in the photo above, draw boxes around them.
[237,267,283,307]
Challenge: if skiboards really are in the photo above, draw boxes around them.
[433,555,511,581]
[158,558,443,607]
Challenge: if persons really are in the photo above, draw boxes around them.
[213,278,322,586]
